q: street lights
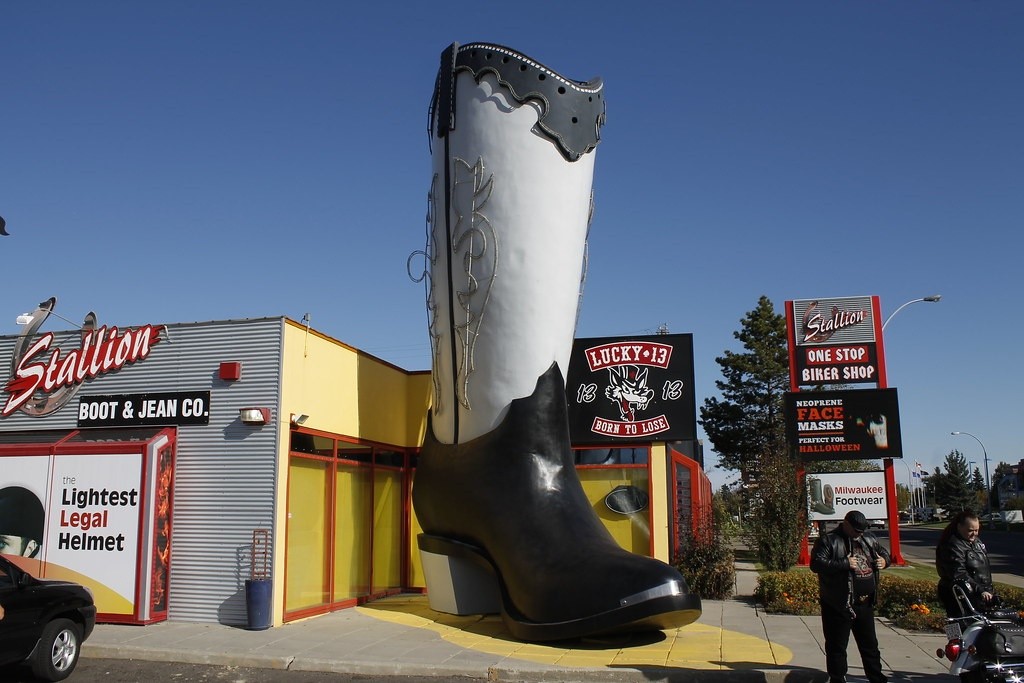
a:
[950,431,993,530]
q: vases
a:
[245,579,272,630]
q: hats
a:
[845,509,869,533]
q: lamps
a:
[15,308,82,330]
[239,407,271,425]
[291,414,310,424]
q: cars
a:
[0,554,97,683]
[896,506,955,524]
[990,512,1002,522]
[867,519,885,530]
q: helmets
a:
[0,485,45,544]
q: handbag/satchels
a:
[843,537,881,608]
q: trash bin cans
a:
[245,579,273,628]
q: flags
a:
[913,462,930,478]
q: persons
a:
[0,487,45,558]
[810,511,891,683]
[936,511,994,633]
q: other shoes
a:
[827,675,848,683]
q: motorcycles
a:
[937,584,1024,683]
